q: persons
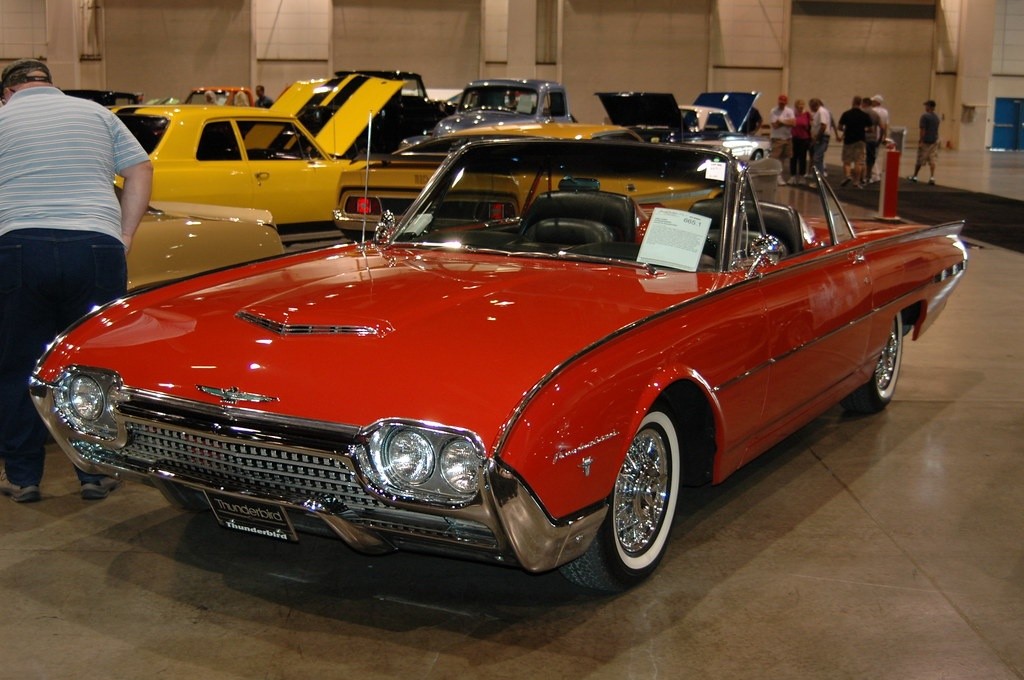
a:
[861,97,887,183]
[0,55,155,505]
[253,84,275,108]
[809,97,842,189]
[767,95,797,186]
[907,100,941,186]
[233,90,251,107]
[837,96,872,192]
[871,94,889,183]
[133,91,146,103]
[204,91,222,105]
[790,98,814,187]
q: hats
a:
[778,95,788,105]
[871,95,884,104]
[923,100,936,107]
[0,58,52,86]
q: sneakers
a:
[0,468,41,502]
[78,475,123,499]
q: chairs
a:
[688,198,804,261]
[516,190,637,244]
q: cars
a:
[63,90,144,106]
[112,180,284,290]
[183,86,254,108]
[102,74,409,234]
[400,77,578,148]
[27,138,967,594]
[334,69,464,155]
[594,90,773,162]
[333,123,721,244]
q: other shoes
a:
[777,175,785,185]
[786,176,796,185]
[839,177,852,187]
[907,175,917,182]
[809,182,818,188]
[928,179,937,185]
[797,177,806,185]
[852,182,863,190]
